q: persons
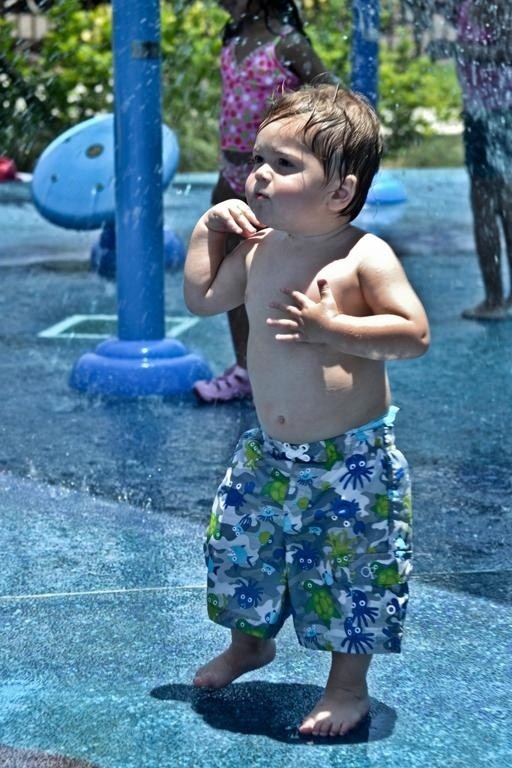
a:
[191,0,330,404]
[402,0,511,320]
[183,86,430,736]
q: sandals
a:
[193,365,250,402]
